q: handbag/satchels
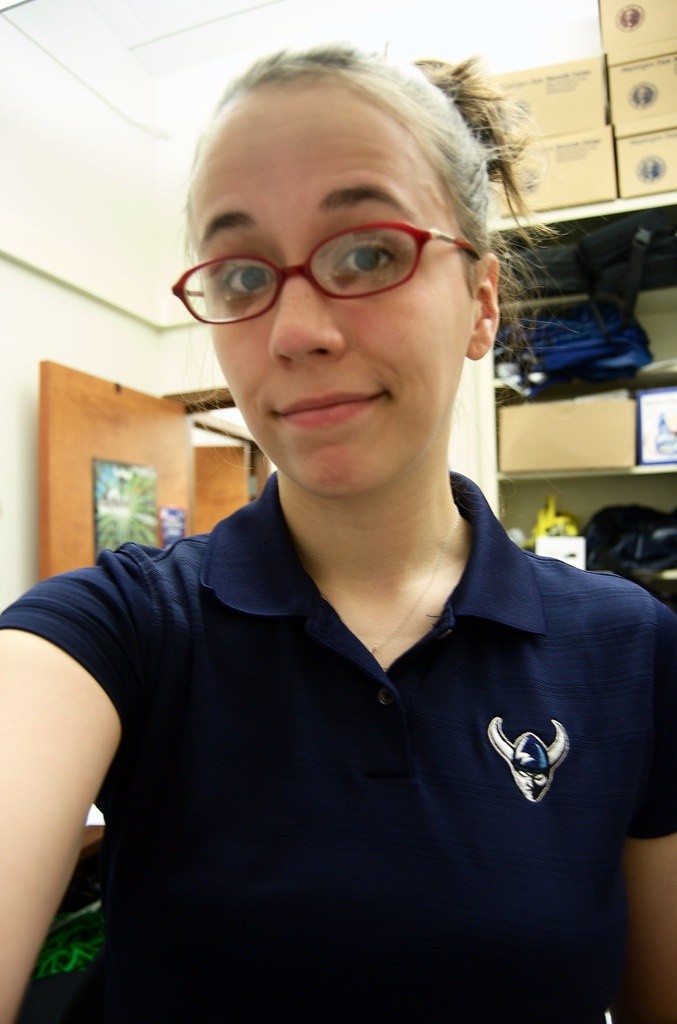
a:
[579,505,677,575]
[499,205,677,333]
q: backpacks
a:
[492,301,652,399]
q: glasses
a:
[172,221,481,325]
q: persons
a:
[0,46,677,1024]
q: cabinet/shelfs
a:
[470,193,677,580]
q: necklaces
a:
[320,505,459,652]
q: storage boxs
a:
[462,0,677,217]
[492,370,677,472]
